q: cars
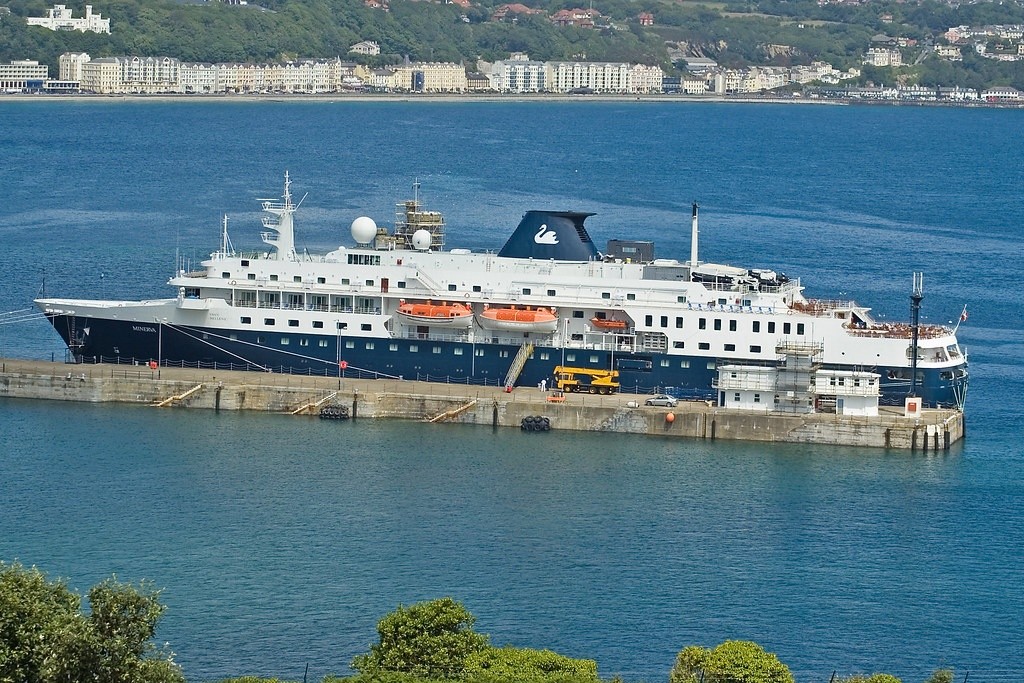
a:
[645,395,679,406]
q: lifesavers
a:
[786,309,794,316]
[566,338,571,345]
[231,280,238,287]
[484,337,491,343]
[463,292,471,298]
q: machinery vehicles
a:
[554,364,620,396]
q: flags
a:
[961,310,968,322]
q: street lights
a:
[469,326,474,385]
[562,318,566,369]
[333,319,339,377]
[153,316,166,369]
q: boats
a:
[739,279,779,290]
[479,309,558,333]
[690,272,736,283]
[32,167,970,412]
[745,269,788,285]
[395,301,474,330]
[590,318,627,329]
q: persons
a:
[541,377,550,391]
